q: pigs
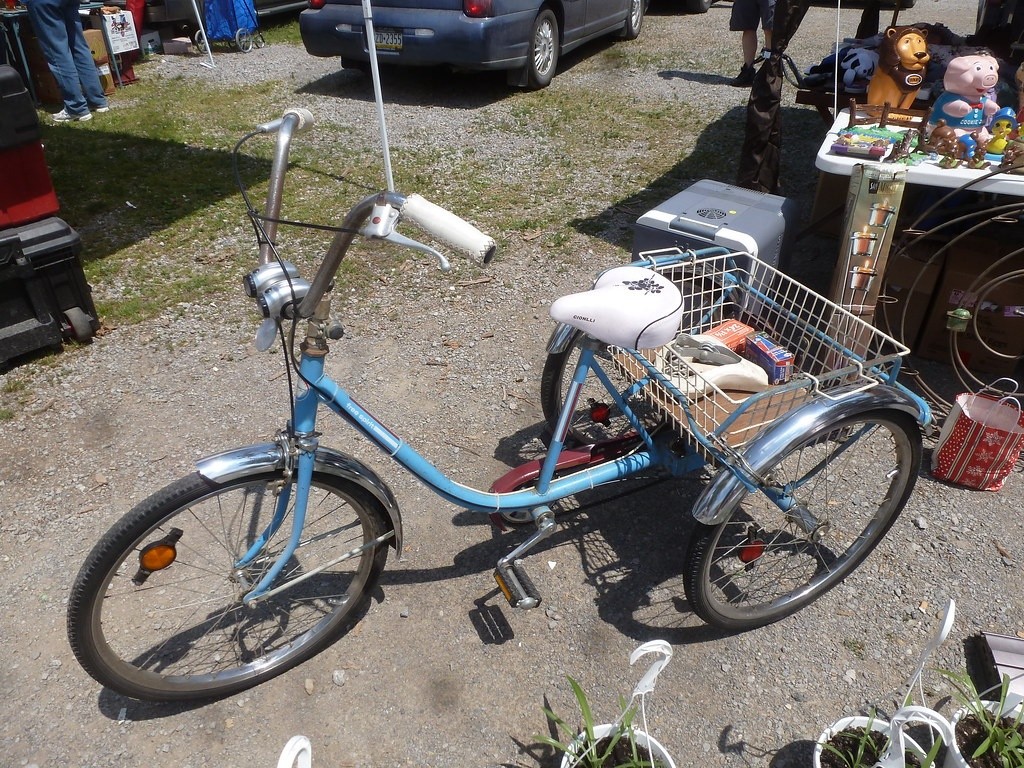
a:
[926,54,999,146]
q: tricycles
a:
[67,107,932,722]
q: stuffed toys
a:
[841,47,880,87]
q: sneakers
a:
[86,103,109,113]
[52,108,92,123]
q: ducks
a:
[986,107,1017,154]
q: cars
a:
[298,0,647,94]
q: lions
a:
[868,27,933,121]
[1000,142,1023,173]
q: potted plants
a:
[932,664,1024,768]
[813,693,936,768]
[531,676,678,768]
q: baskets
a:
[608,252,912,472]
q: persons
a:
[730,0,777,87]
[20,0,109,122]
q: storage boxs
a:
[76,8,139,54]
[916,244,1024,378]
[829,161,909,382]
[162,37,192,55]
[29,28,109,72]
[702,319,754,353]
[139,29,163,57]
[971,630,1024,702]
[870,238,944,353]
[631,180,786,326]
[34,62,116,104]
[744,330,793,386]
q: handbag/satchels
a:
[930,378,1024,492]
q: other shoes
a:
[737,63,755,86]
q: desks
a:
[815,106,1023,195]
[0,2,123,107]
